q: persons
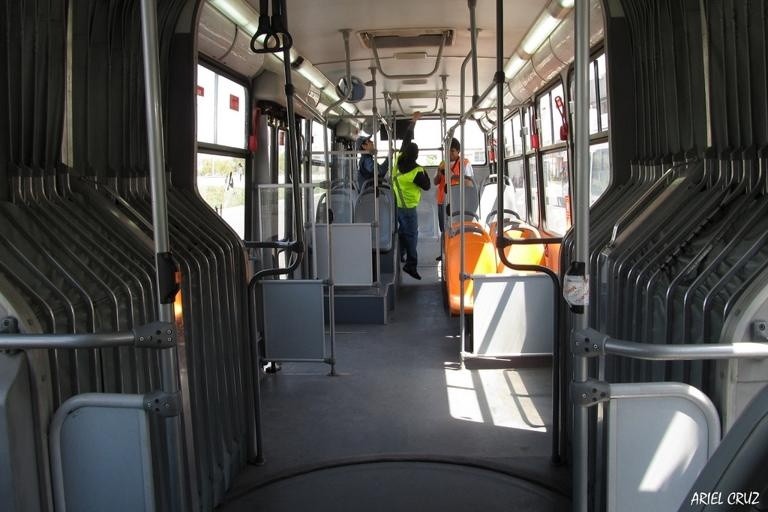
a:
[432,136,474,262]
[354,134,393,193]
[390,110,431,280]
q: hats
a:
[356,135,372,151]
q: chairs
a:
[318,177,394,253]
[444,173,547,315]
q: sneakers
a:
[436,257,442,260]
[403,262,421,280]
[401,255,405,262]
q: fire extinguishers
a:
[489,138,495,160]
